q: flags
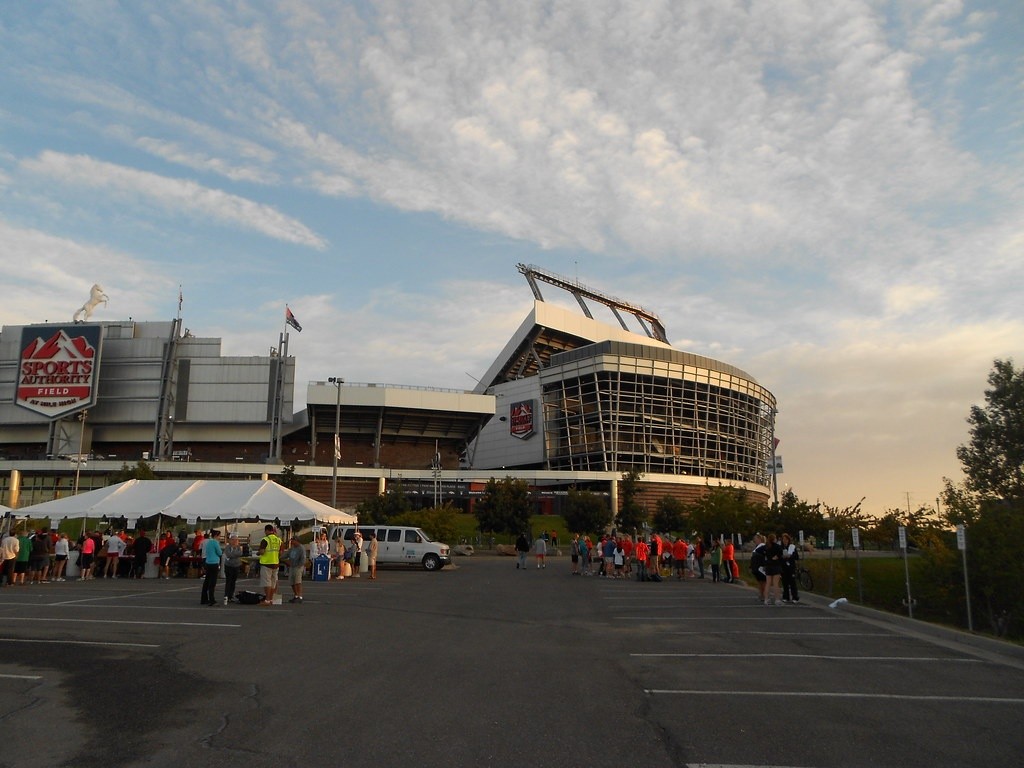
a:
[286,307,302,332]
[178,288,183,311]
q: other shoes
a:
[792,599,799,604]
[762,599,773,605]
[523,567,527,569]
[516,562,520,568]
[5,572,170,586]
[572,569,633,579]
[224,597,235,605]
[352,573,361,578]
[260,600,273,606]
[542,564,545,568]
[336,575,344,580]
[537,565,540,568]
[369,576,376,579]
[698,575,704,579]
[782,599,788,602]
[775,599,785,605]
[288,596,303,603]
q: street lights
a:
[328,377,344,551]
[771,405,778,508]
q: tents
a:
[0,504,27,531]
[0,479,358,581]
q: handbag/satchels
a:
[154,557,160,566]
[343,551,352,560]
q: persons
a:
[514,529,739,584]
[129,530,152,579]
[223,536,243,605]
[334,537,345,579]
[2,527,70,586]
[309,532,328,580]
[76,528,135,580]
[351,532,363,577]
[748,533,800,605]
[157,527,187,580]
[192,528,223,606]
[365,533,378,578]
[256,525,304,607]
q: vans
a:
[327,526,451,571]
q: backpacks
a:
[235,590,265,605]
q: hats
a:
[140,530,145,536]
[289,535,300,542]
[354,532,361,535]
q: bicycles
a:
[793,558,814,592]
